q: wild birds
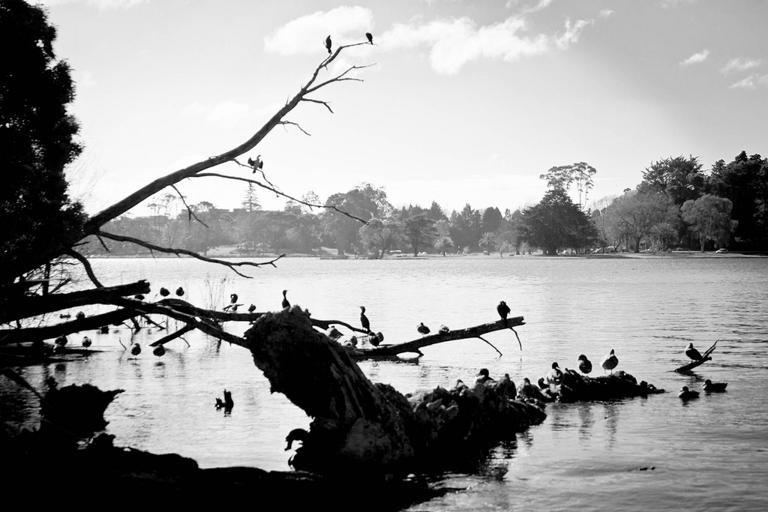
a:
[684,342,702,364]
[79,335,93,350]
[280,289,289,308]
[416,322,448,335]
[228,293,257,312]
[496,301,511,321]
[54,332,69,348]
[160,287,184,298]
[454,349,620,407]
[130,343,164,362]
[327,305,380,349]
[75,310,84,321]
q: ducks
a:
[678,379,729,400]
